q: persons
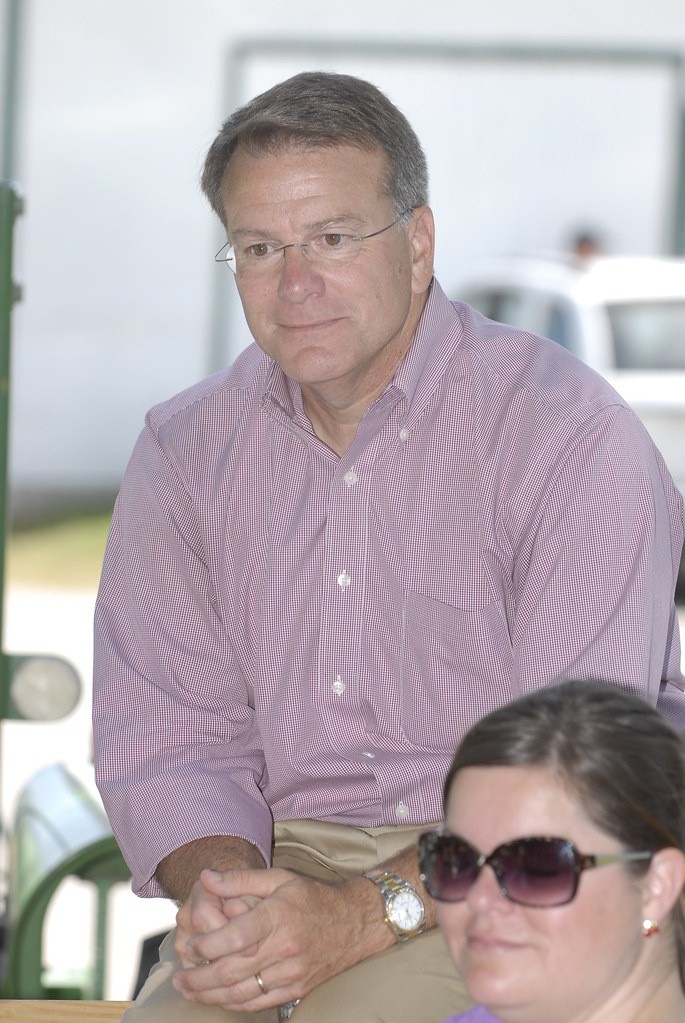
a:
[434,682,685,1023]
[92,75,685,1023]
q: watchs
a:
[364,868,425,943]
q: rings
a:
[255,973,267,994]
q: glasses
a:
[216,211,404,275]
[419,828,652,906]
